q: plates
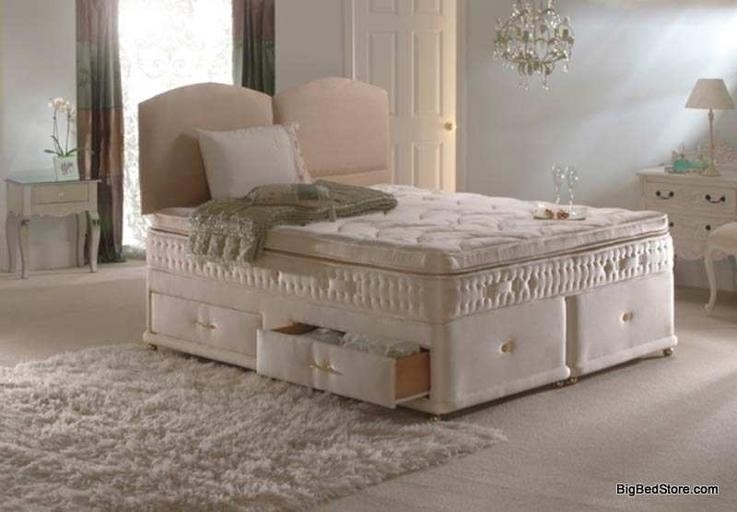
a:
[535,207,588,220]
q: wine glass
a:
[551,165,581,215]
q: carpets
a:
[0,332,511,511]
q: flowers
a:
[44,93,81,160]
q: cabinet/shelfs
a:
[4,173,102,277]
[634,165,737,263]
[144,285,431,412]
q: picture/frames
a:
[56,153,81,181]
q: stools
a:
[702,221,736,314]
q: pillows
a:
[192,119,309,204]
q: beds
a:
[138,70,678,420]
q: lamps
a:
[488,0,576,91]
[685,78,736,178]
